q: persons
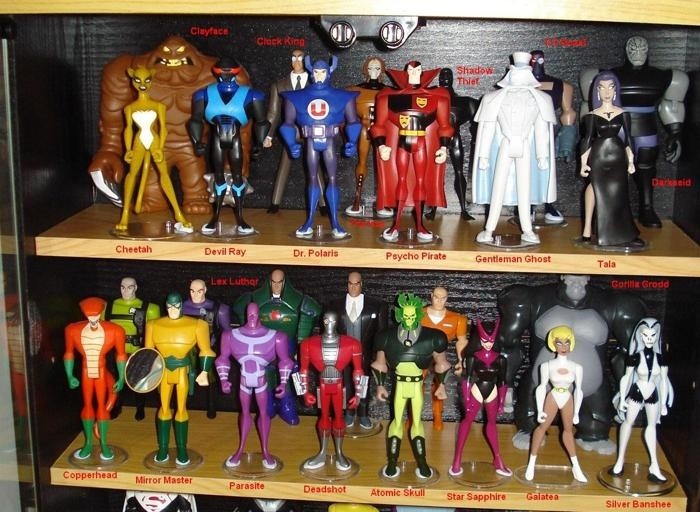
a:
[62,274,678,512]
[187,36,690,250]
[63,274,673,482]
[86,35,691,255]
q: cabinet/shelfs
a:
[1,3,700,511]
